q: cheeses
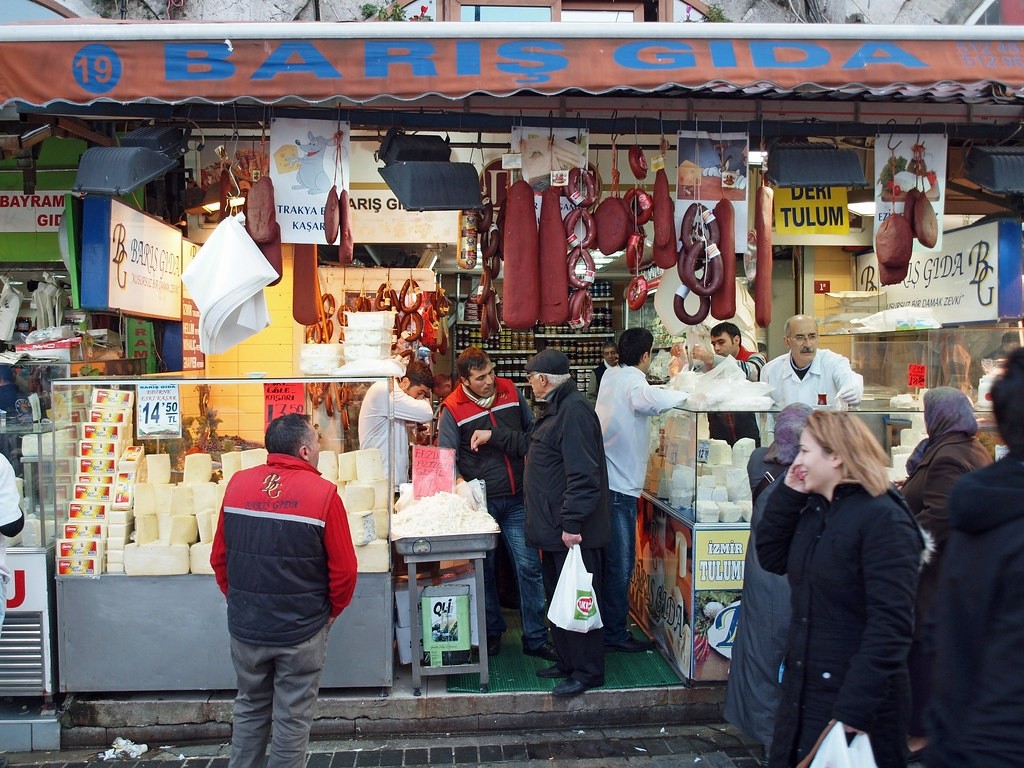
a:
[886,388,931,482]
[122,448,268,574]
[316,449,390,573]
[648,412,756,524]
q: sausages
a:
[306,279,450,429]
[564,167,600,328]
[673,203,725,326]
[476,194,504,339]
[625,147,654,312]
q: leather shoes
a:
[537,662,571,678]
[603,632,657,653]
[552,676,606,697]
[472,635,499,656]
[522,639,560,662]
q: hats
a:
[524,349,570,375]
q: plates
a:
[708,601,740,658]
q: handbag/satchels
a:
[547,542,607,631]
[797,721,878,768]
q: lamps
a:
[764,137,870,189]
[963,143,1024,193]
[71,119,192,199]
[376,126,486,211]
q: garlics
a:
[703,601,724,617]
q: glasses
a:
[788,334,818,342]
[525,371,539,383]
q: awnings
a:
[0,14,1024,117]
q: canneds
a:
[456,279,613,391]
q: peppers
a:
[694,629,709,662]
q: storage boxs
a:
[15,342,79,363]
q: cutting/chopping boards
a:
[880,171,939,202]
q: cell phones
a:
[796,467,804,481]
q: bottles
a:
[977,375,995,407]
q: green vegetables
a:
[695,591,743,634]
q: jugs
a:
[907,145,933,176]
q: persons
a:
[469,347,611,697]
[761,314,866,410]
[721,349,1024,768]
[0,365,29,476]
[436,345,558,662]
[355,358,434,494]
[596,326,688,653]
[0,450,24,768]
[210,413,359,768]
[434,373,462,397]
[670,322,766,448]
[588,341,619,410]
